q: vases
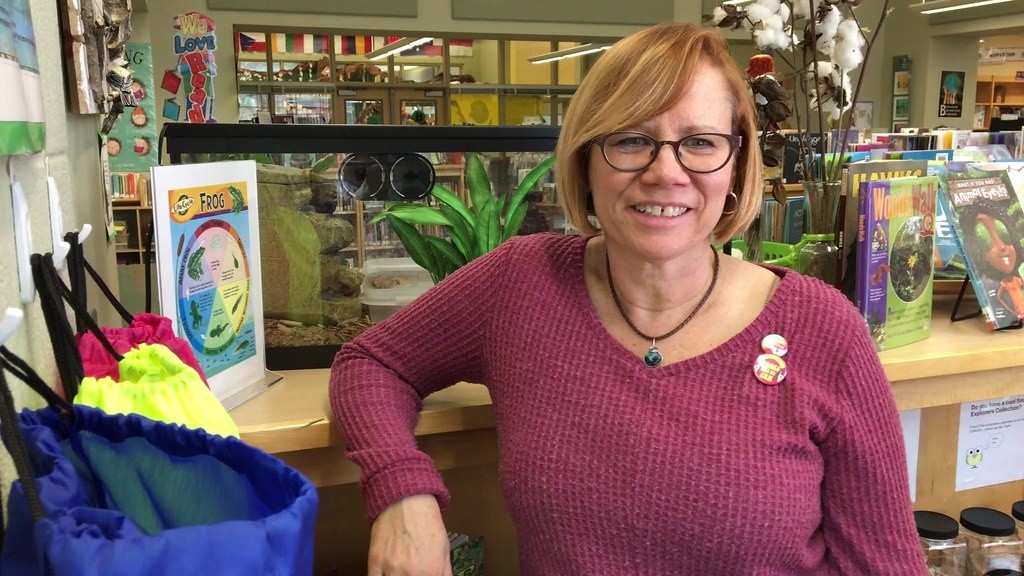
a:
[800,179,842,291]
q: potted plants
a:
[364,156,559,287]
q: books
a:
[755,128,1024,354]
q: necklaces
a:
[606,243,719,367]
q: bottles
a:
[913,500,1024,576]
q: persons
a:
[330,22,931,576]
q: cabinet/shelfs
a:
[104,175,157,264]
[972,75,1023,134]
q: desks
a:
[220,279,1022,576]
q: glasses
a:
[592,130,742,174]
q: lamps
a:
[527,43,614,65]
[364,37,433,61]
[909,1,1012,16]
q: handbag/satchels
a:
[1,344,318,576]
[63,231,212,393]
[28,252,242,440]
